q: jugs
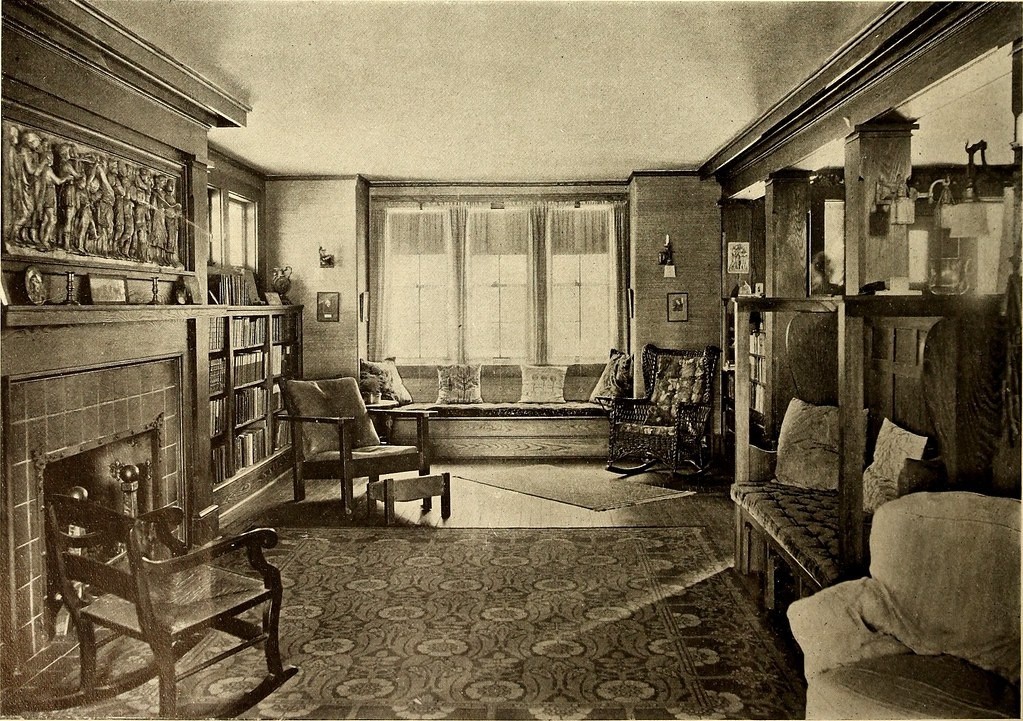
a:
[924,256,972,296]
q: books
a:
[206,272,251,307]
[232,316,269,348]
[207,315,227,350]
[272,344,296,378]
[208,356,227,394]
[272,382,286,412]
[274,416,294,452]
[235,387,267,428]
[271,315,299,342]
[210,397,226,436]
[211,440,230,484]
[232,420,268,476]
[233,349,268,387]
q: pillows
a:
[519,363,566,404]
[862,417,929,512]
[361,358,414,404]
[435,362,483,405]
[776,398,868,489]
[588,347,630,407]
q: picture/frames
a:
[667,292,689,322]
[318,292,339,322]
[86,272,128,306]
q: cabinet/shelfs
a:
[204,305,300,526]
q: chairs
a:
[277,376,435,515]
[46,465,299,719]
[785,491,1021,720]
[595,341,722,476]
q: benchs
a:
[732,481,872,612]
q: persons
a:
[10,130,182,266]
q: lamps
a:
[319,245,334,268]
[875,137,1022,237]
[659,236,677,280]
